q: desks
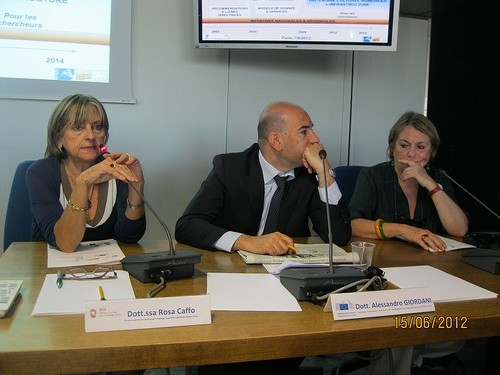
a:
[0,236,500,375]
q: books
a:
[237,243,366,264]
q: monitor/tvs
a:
[193,0,401,53]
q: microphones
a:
[279,149,378,303]
[430,164,500,274]
[99,144,202,284]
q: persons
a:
[175,99,353,375]
[26,93,147,253]
[347,110,469,375]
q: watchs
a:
[430,183,444,195]
[316,167,336,181]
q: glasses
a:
[62,264,118,280]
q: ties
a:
[263,175,291,234]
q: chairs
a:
[4,160,38,251]
[330,166,371,202]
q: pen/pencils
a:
[286,244,300,252]
[98,285,106,300]
[57,271,63,288]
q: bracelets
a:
[375,219,387,240]
[127,198,145,209]
[68,199,92,210]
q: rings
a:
[422,234,428,239]
[109,164,114,168]
